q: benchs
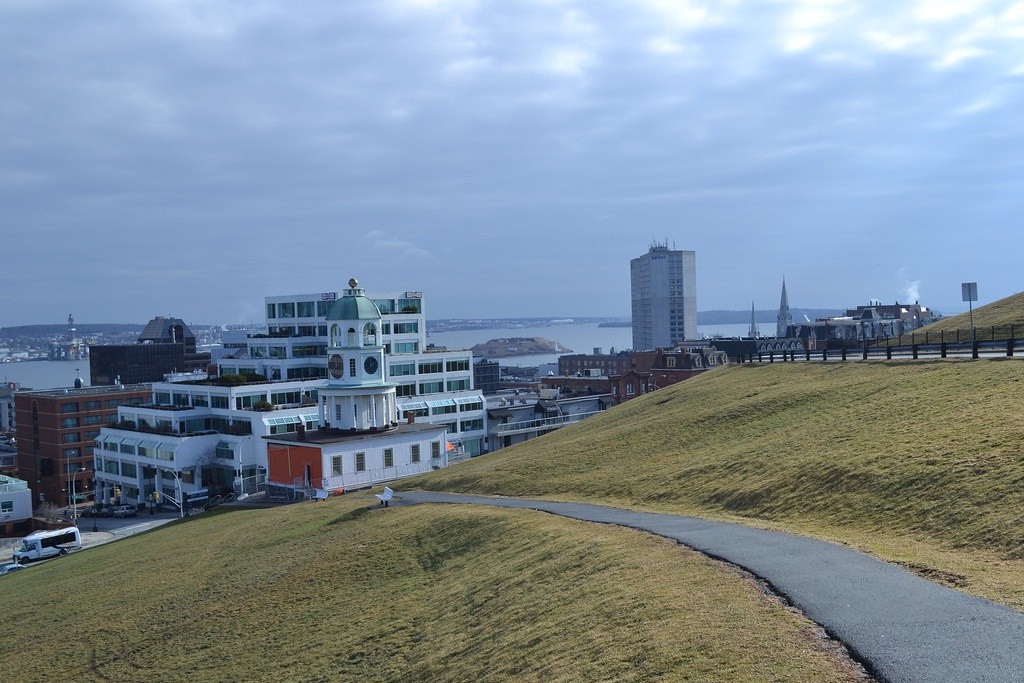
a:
[312,490,329,502]
[375,486,394,508]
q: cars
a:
[0,563,27,576]
[113,505,137,519]
[96,508,113,517]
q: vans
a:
[12,527,82,561]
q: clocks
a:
[363,356,379,375]
[328,354,343,380]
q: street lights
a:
[73,467,86,527]
[239,436,253,496]
[164,468,184,518]
